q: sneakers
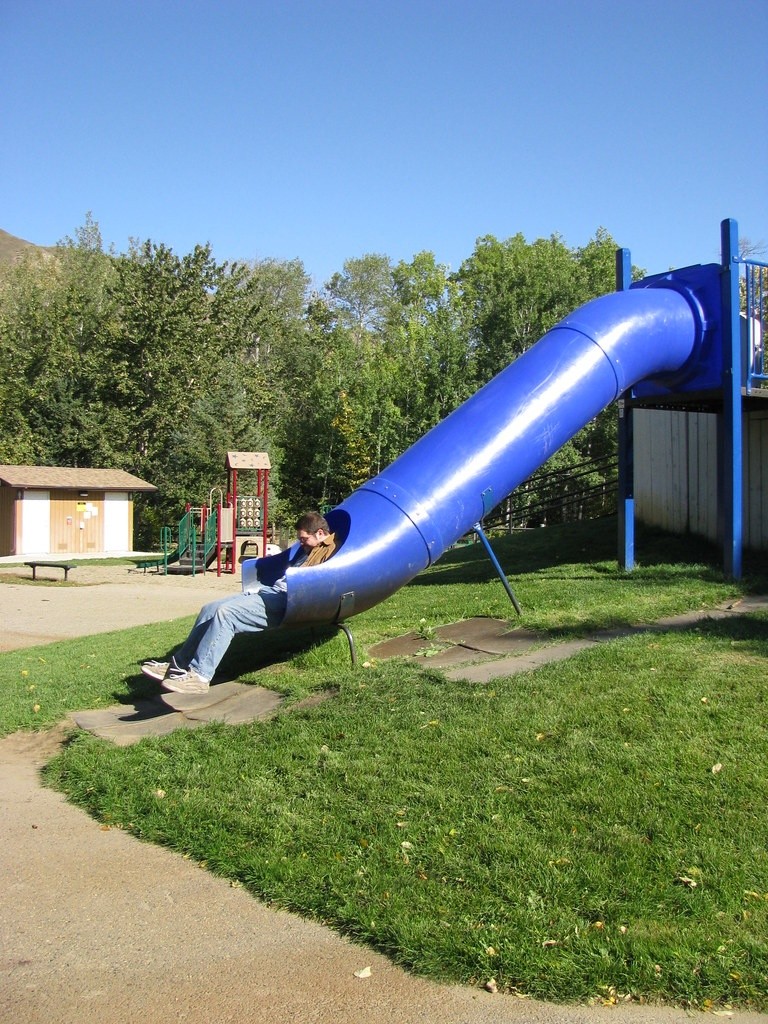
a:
[141,656,210,694]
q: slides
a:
[238,263,734,639]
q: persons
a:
[140,512,344,695]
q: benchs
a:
[24,561,77,581]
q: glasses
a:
[300,528,323,543]
